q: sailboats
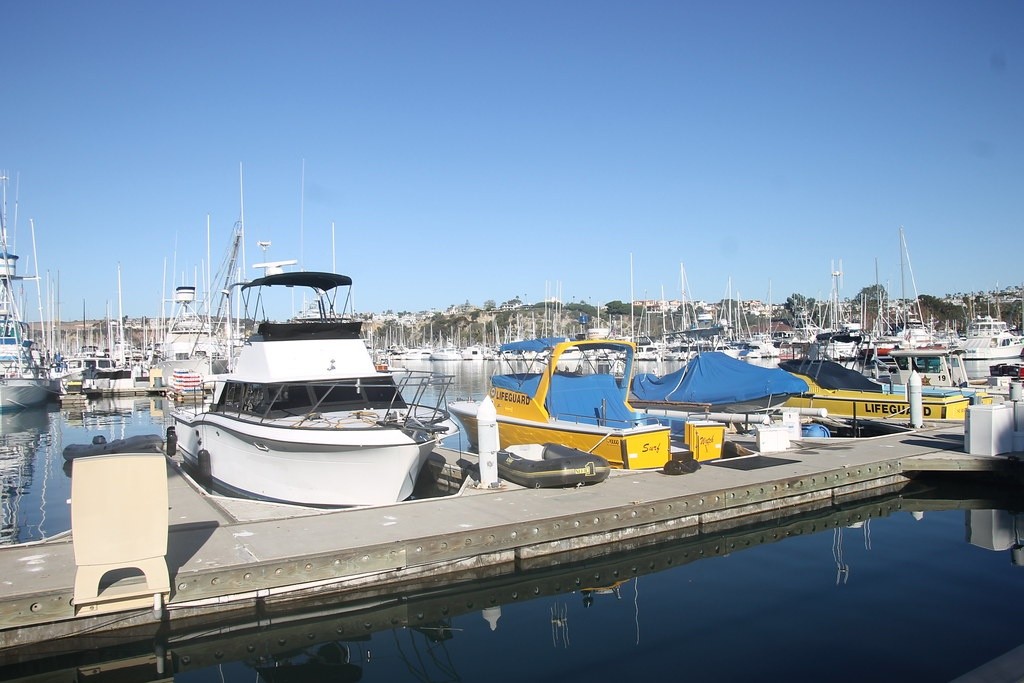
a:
[0,160,969,414]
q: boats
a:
[627,351,810,416]
[446,333,726,476]
[777,357,994,420]
[500,442,611,489]
[167,161,459,510]
[953,297,1024,360]
